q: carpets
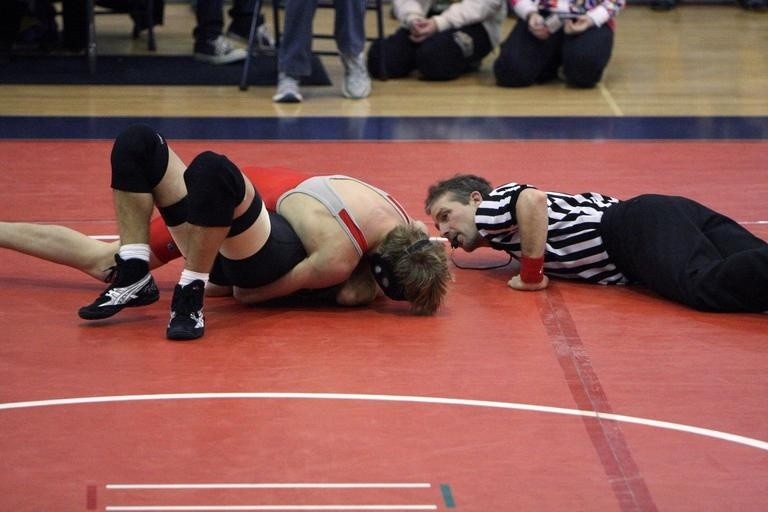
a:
[3,48,333,86]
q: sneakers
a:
[274,72,302,102]
[338,51,372,99]
[194,35,247,65]
[79,254,160,320]
[167,279,205,339]
[227,20,274,47]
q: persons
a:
[366,0,508,80]
[271,0,373,102]
[0,166,448,307]
[192,0,276,65]
[79,124,384,340]
[1,1,96,64]
[492,1,627,87]
[424,175,767,310]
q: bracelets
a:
[519,255,544,284]
